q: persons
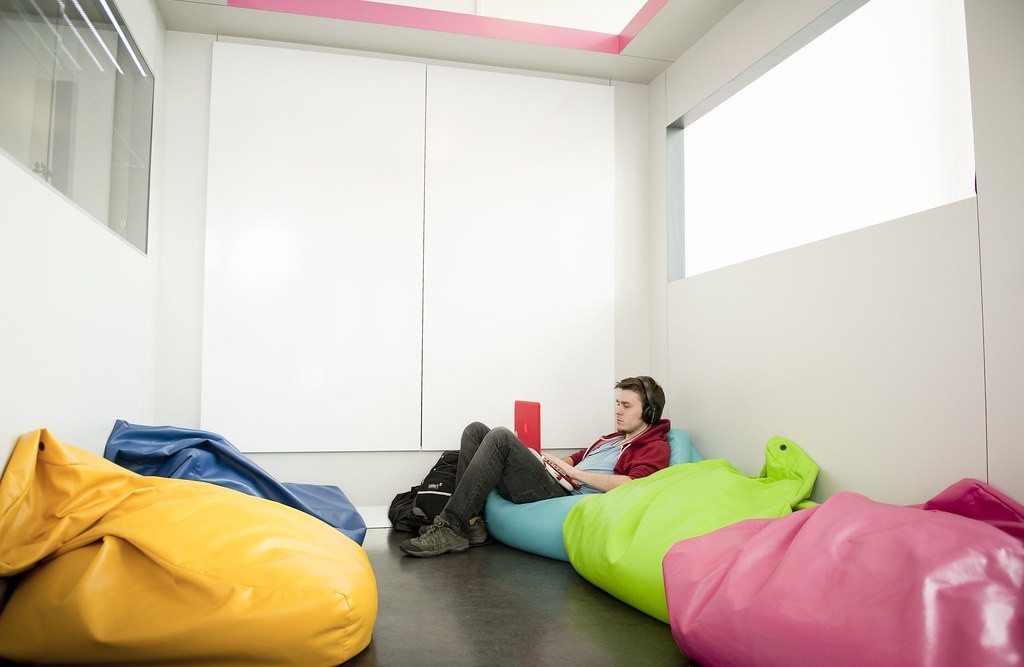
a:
[399,375,670,556]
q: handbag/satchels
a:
[388,485,421,532]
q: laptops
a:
[513,400,581,489]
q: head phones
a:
[637,375,663,425]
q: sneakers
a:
[399,515,470,557]
[467,519,487,544]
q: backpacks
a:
[412,449,459,525]
